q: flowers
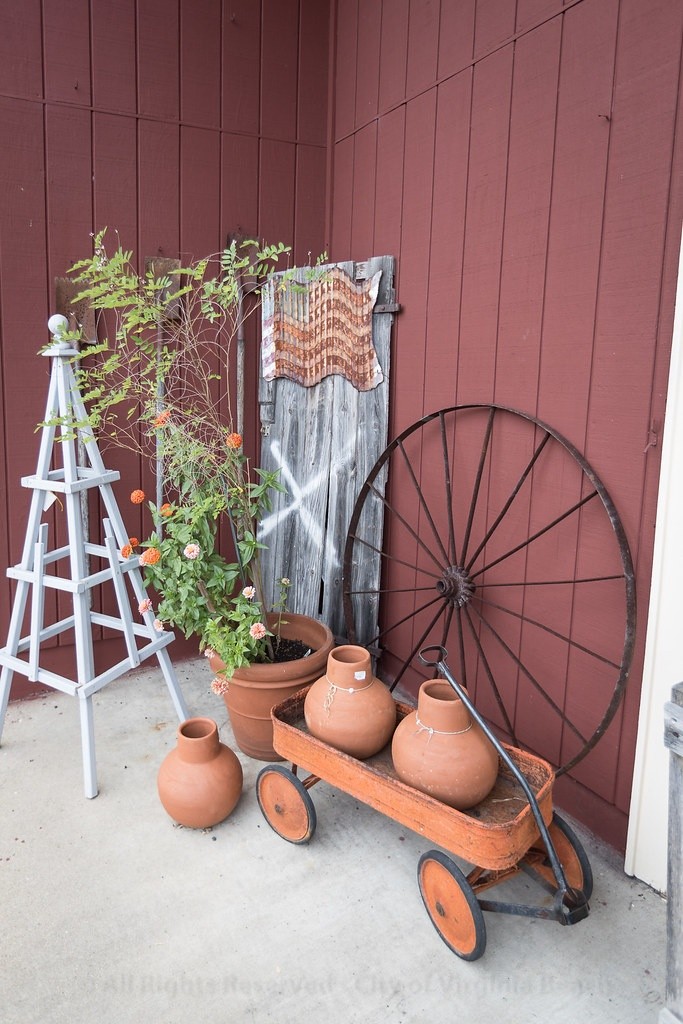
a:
[113,397,269,696]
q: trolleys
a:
[255,683,593,964]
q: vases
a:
[155,719,245,831]
[392,680,498,811]
[305,642,398,759]
[208,610,336,766]
